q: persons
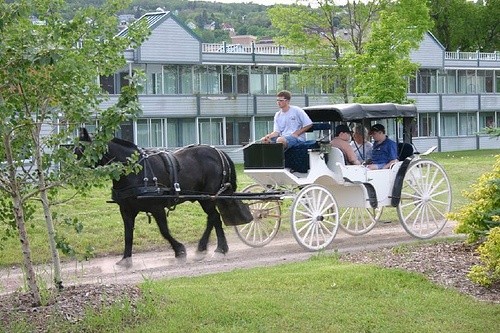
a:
[330,124,362,164]
[260,90,314,154]
[366,123,398,170]
[350,125,373,163]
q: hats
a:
[368,124,384,134]
[336,125,353,133]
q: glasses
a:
[277,98,287,102]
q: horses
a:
[74,127,237,266]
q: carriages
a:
[63,101,452,269]
